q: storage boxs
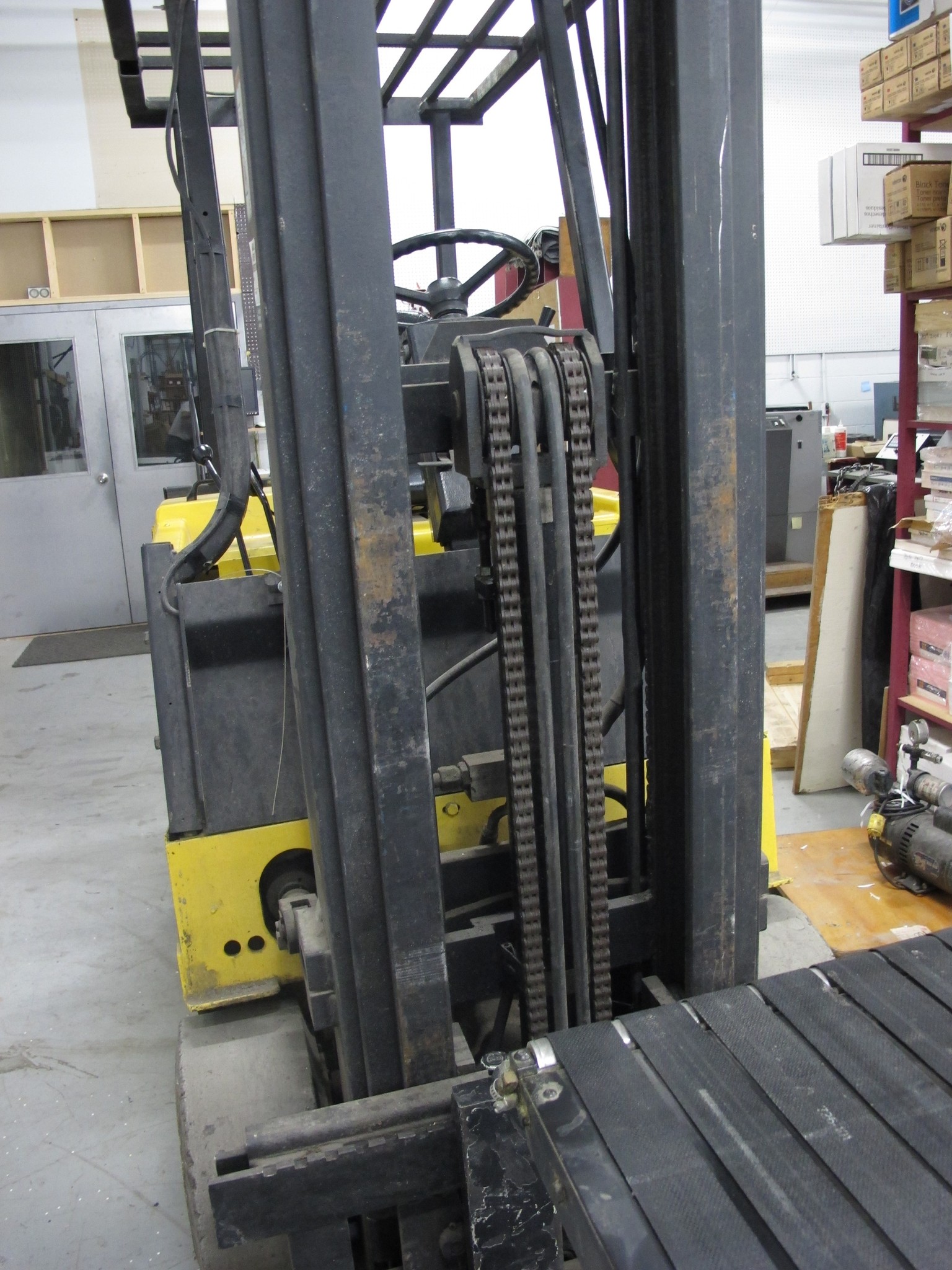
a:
[859,0,952,121]
[819,143,952,293]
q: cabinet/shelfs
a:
[887,113,952,807]
